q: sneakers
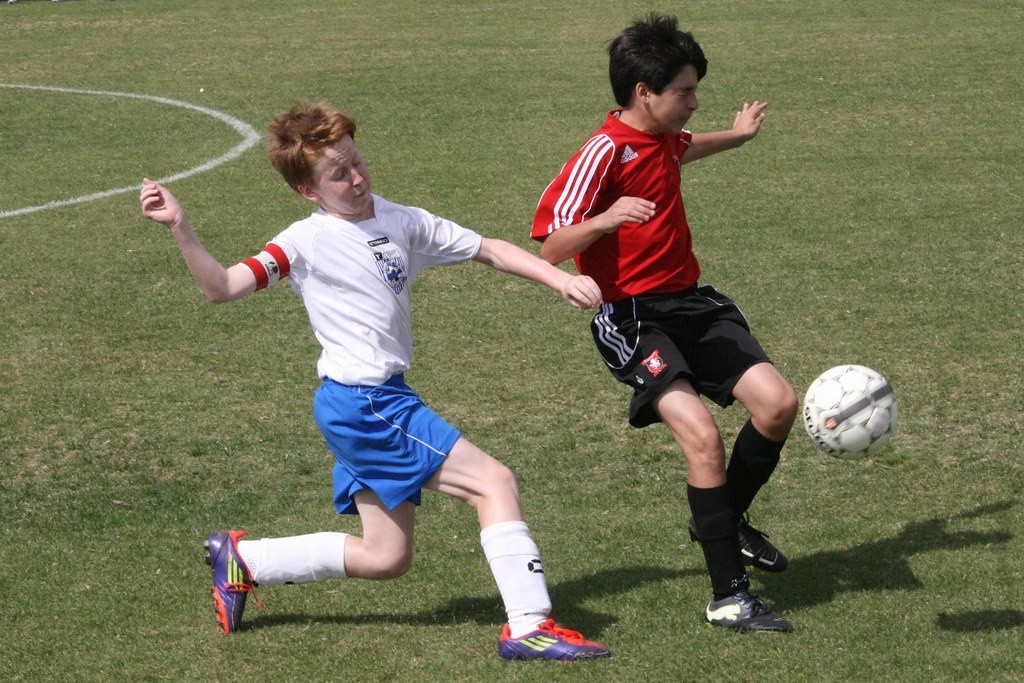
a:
[203,531,262,635]
[496,619,611,664]
[688,511,789,572]
[704,591,794,633]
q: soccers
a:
[803,361,897,461]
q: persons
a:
[138,102,612,661]
[529,13,797,637]
